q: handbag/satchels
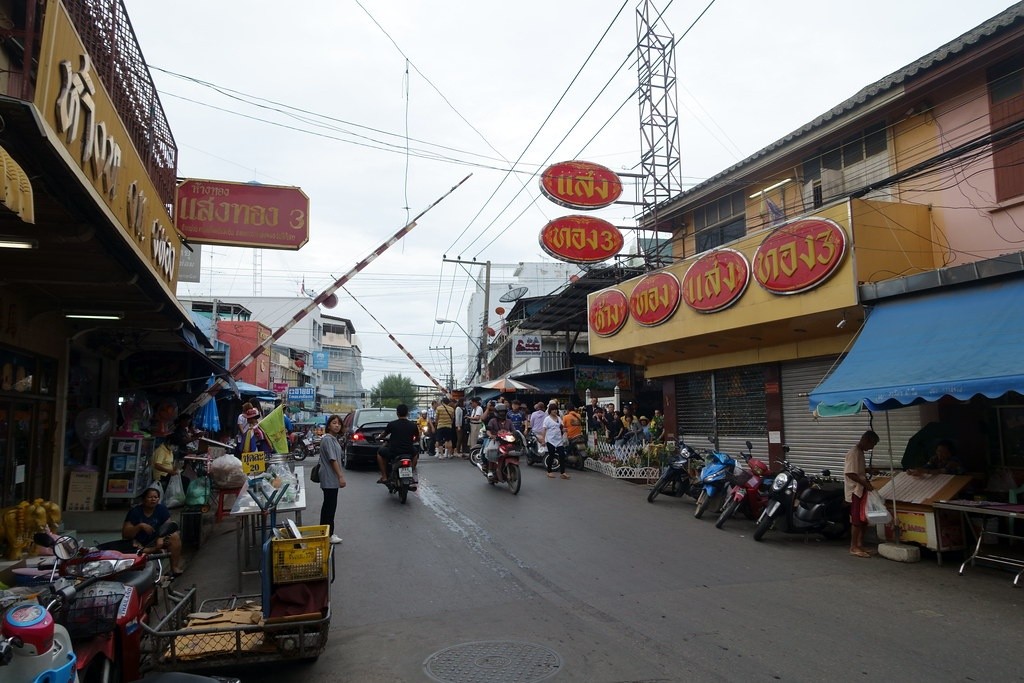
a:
[209,454,247,488]
[310,463,321,484]
[484,437,498,462]
[185,476,210,505]
[149,481,164,504]
[164,473,186,508]
[860,488,893,525]
[562,432,570,447]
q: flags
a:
[259,405,288,453]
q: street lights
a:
[435,317,487,384]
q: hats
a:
[638,416,649,422]
[242,409,260,419]
[534,402,546,412]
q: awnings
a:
[234,380,276,396]
[799,275,1024,545]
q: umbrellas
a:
[474,377,540,399]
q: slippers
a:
[377,478,387,483]
[409,477,415,484]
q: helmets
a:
[493,403,508,419]
[421,410,428,419]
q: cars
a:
[337,408,399,471]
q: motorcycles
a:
[375,436,419,505]
[476,428,525,495]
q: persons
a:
[584,397,665,445]
[121,488,183,575]
[173,413,208,473]
[542,404,570,478]
[375,404,420,483]
[417,410,427,431]
[317,415,346,544]
[455,398,583,472]
[275,399,293,431]
[906,440,981,499]
[241,409,272,454]
[150,433,189,490]
[843,431,879,558]
[237,402,252,441]
[435,398,457,459]
[427,401,438,456]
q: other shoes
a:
[488,472,494,479]
[849,549,870,558]
[419,444,471,460]
[858,547,872,553]
[329,534,343,543]
[547,472,556,478]
[560,473,570,479]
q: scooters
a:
[647,428,777,529]
[752,444,851,544]
[0,519,244,683]
[469,422,589,474]
[281,425,324,462]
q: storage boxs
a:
[271,524,330,582]
[65,470,101,513]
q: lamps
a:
[64,309,128,320]
[0,234,39,249]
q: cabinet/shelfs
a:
[101,435,156,511]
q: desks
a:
[229,462,307,575]
[936,499,1024,588]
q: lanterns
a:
[495,307,504,315]
[489,328,494,336]
[296,360,304,368]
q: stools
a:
[216,486,242,524]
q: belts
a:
[470,422,483,424]
